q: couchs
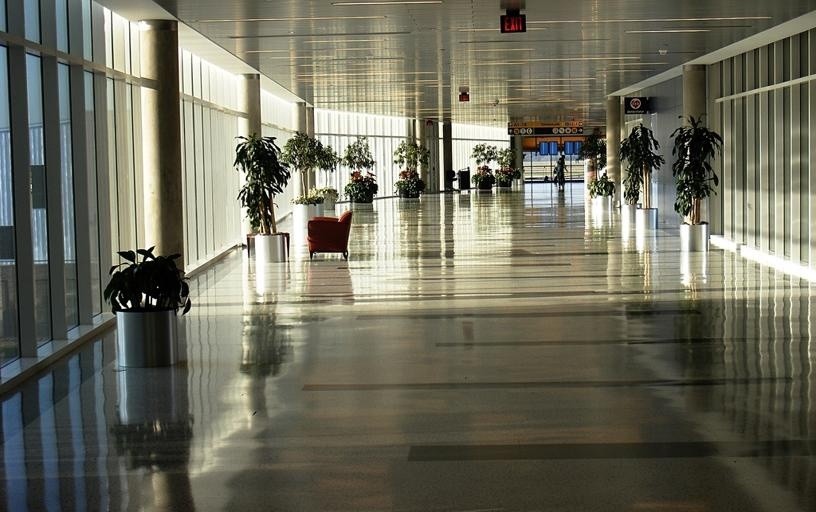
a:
[307,208,353,261]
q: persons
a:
[555,155,569,192]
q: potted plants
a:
[233,132,290,261]
[281,129,520,220]
[586,170,618,211]
[616,122,661,230]
[666,112,726,253]
[102,246,193,369]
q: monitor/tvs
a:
[564,141,573,155]
[549,141,558,155]
[540,142,548,155]
[574,141,583,155]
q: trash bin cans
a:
[458,167,470,190]
